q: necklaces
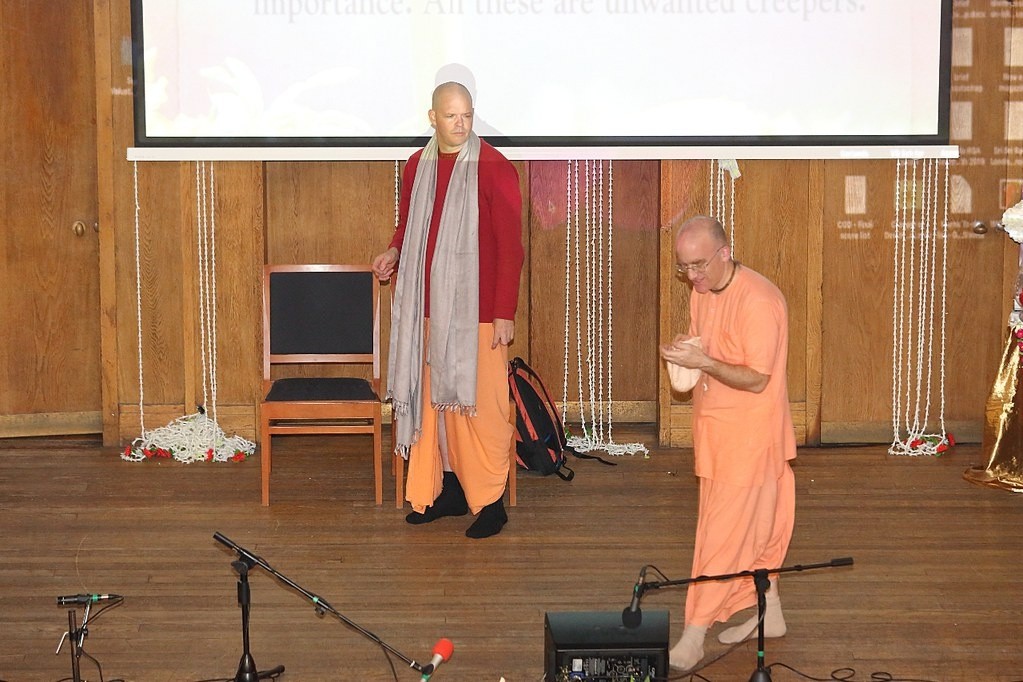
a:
[709,259,738,292]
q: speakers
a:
[543,611,671,682]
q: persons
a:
[660,214,797,669]
[370,82,525,539]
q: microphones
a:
[420,638,454,682]
[58,593,119,605]
[621,566,647,628]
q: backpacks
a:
[507,356,568,475]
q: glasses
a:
[674,243,726,273]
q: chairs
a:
[391,402,519,507]
[259,262,384,506]
